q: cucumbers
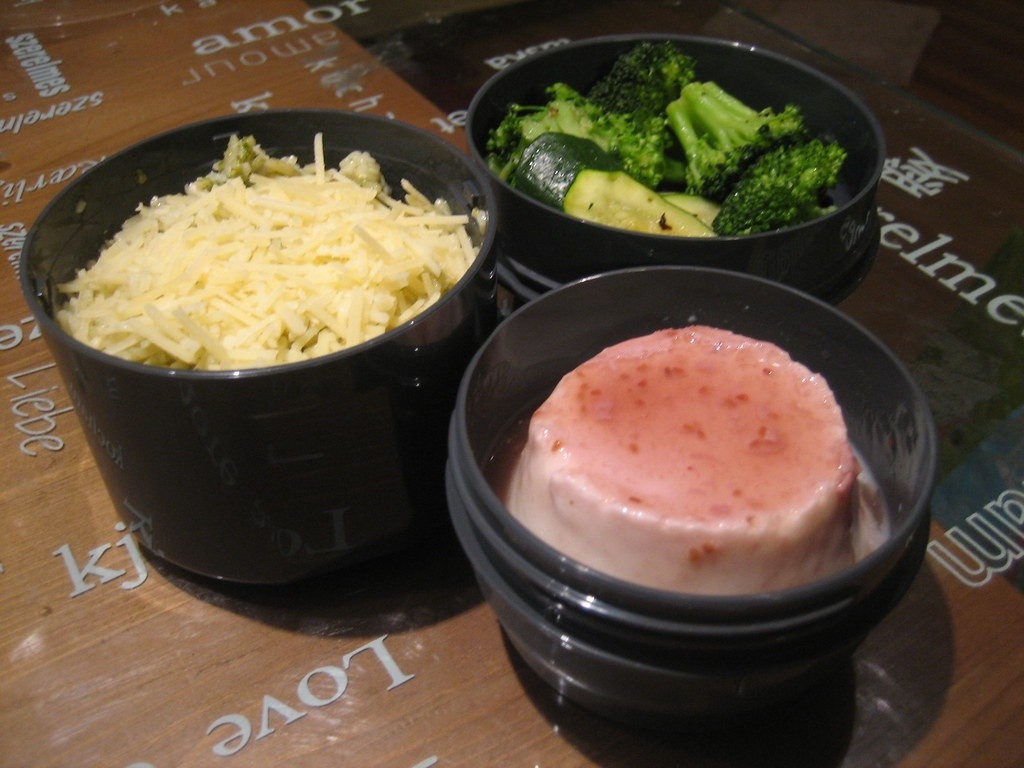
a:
[514,133,718,237]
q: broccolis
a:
[486,39,848,238]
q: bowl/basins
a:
[20,107,490,586]
[464,37,881,300]
[445,267,938,721]
